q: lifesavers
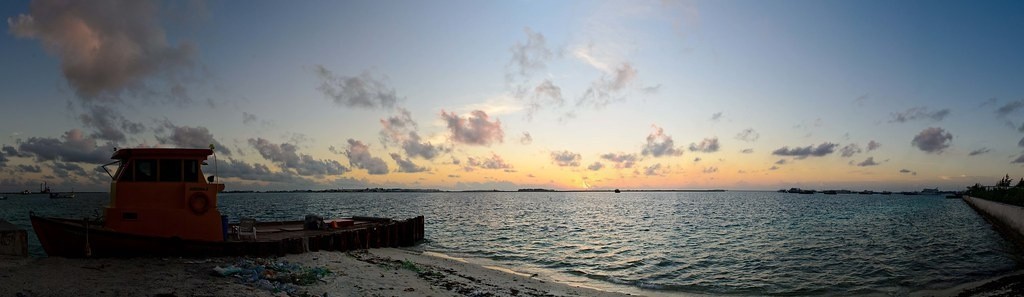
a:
[189,192,210,213]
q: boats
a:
[0,197,8,200]
[27,147,426,258]
[49,191,75,198]
[24,190,31,195]
